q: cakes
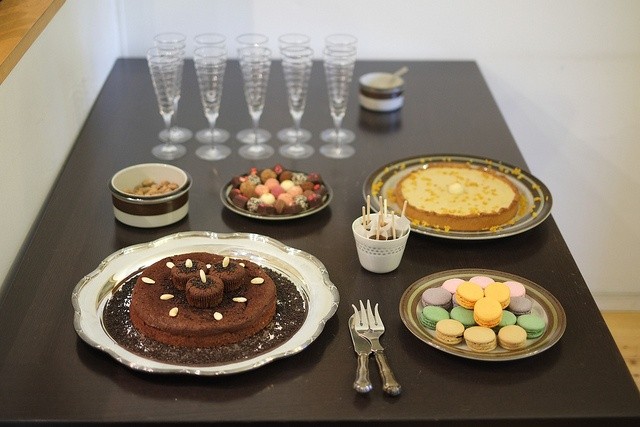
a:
[396,165,520,231]
[129,252,278,348]
[229,163,327,215]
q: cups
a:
[352,214,412,272]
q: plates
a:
[363,154,553,241]
[71,230,341,383]
[398,266,569,361]
[220,167,333,220]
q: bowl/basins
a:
[358,71,406,113]
[110,163,192,231]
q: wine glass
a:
[236,31,272,145]
[278,34,311,142]
[321,45,356,159]
[149,31,191,144]
[237,47,274,161]
[147,48,185,160]
[321,34,356,143]
[195,47,231,161]
[193,28,228,143]
[281,46,316,160]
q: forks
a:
[352,298,403,396]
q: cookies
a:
[420,276,545,353]
